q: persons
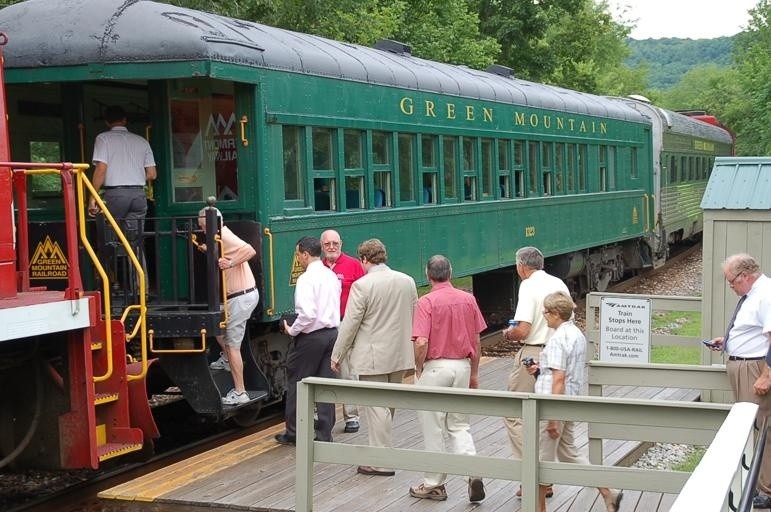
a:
[314,229,361,432]
[525,292,624,512]
[330,237,418,477]
[707,254,771,508]
[502,246,573,498]
[196,206,259,406]
[408,255,488,502]
[285,142,329,194]
[87,105,158,315]
[275,235,343,447]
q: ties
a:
[720,295,747,356]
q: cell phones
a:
[703,340,719,348]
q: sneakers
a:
[468,476,485,501]
[410,483,447,501]
[221,388,250,405]
[275,434,296,444]
[517,484,553,498]
[210,351,231,372]
[358,465,395,476]
[604,489,623,512]
[345,421,359,432]
[753,489,771,509]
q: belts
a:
[519,343,545,348]
[227,285,257,299]
[103,185,144,190]
[729,355,765,361]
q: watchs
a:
[228,259,234,269]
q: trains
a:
[0,1,737,473]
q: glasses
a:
[728,271,744,285]
[325,242,340,246]
